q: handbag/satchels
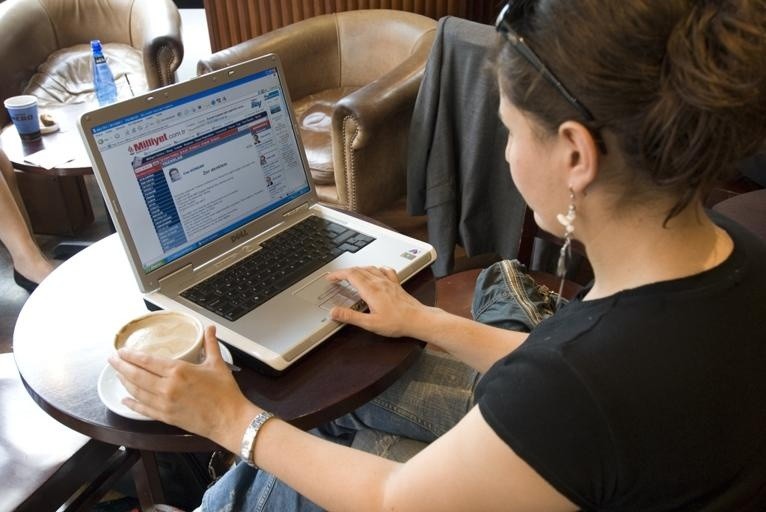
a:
[471,259,570,333]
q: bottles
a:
[89,40,118,109]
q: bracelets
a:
[239,410,278,469]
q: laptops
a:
[76,51,437,371]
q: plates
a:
[97,341,234,421]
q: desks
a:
[13,208,436,451]
[0,106,116,257]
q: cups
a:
[4,95,42,144]
[114,311,205,361]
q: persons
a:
[259,155,267,167]
[106,0,766,512]
[168,167,182,182]
[252,132,262,146]
[264,176,274,187]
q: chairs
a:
[406,17,594,269]
[0,0,183,128]
[197,9,438,216]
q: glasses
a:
[494,0,609,156]
